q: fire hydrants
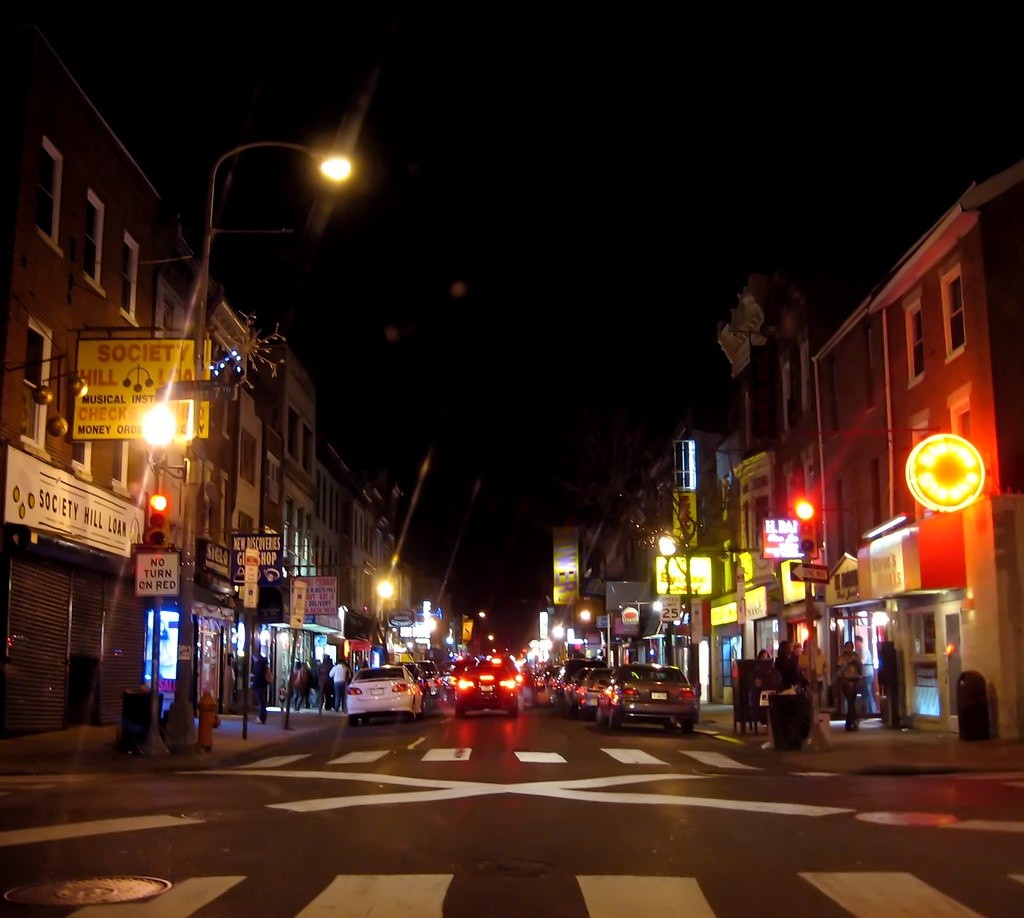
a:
[194,690,221,754]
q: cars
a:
[396,651,614,715]
[349,664,424,727]
[454,664,522,716]
[596,663,701,734]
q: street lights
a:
[168,142,352,745]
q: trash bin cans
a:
[116,685,165,754]
[957,669,991,741]
[766,693,812,753]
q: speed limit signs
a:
[660,595,681,621]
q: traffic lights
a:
[145,492,172,549]
[794,496,817,561]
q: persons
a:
[775,640,827,707]
[252,653,267,724]
[287,658,353,711]
[757,649,770,659]
[836,641,862,729]
[855,636,877,712]
[356,655,369,669]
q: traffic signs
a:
[790,562,831,585]
